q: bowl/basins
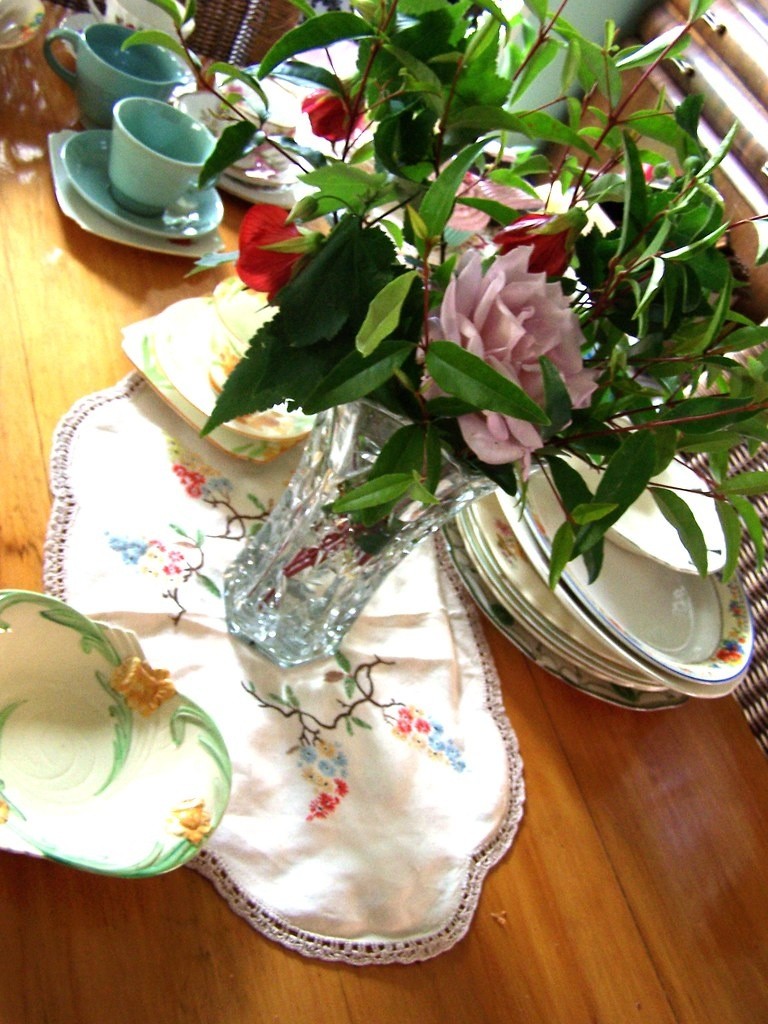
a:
[0,0,45,49]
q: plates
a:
[57,12,196,103]
[174,91,323,211]
[119,318,313,465]
[440,414,755,713]
[148,296,316,443]
[0,587,232,879]
[48,129,223,258]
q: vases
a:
[227,395,500,667]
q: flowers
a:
[120,1,768,608]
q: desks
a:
[0,1,768,1024]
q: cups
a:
[204,280,279,414]
[88,0,194,45]
[219,81,297,171]
[42,23,189,129]
[108,96,221,219]
